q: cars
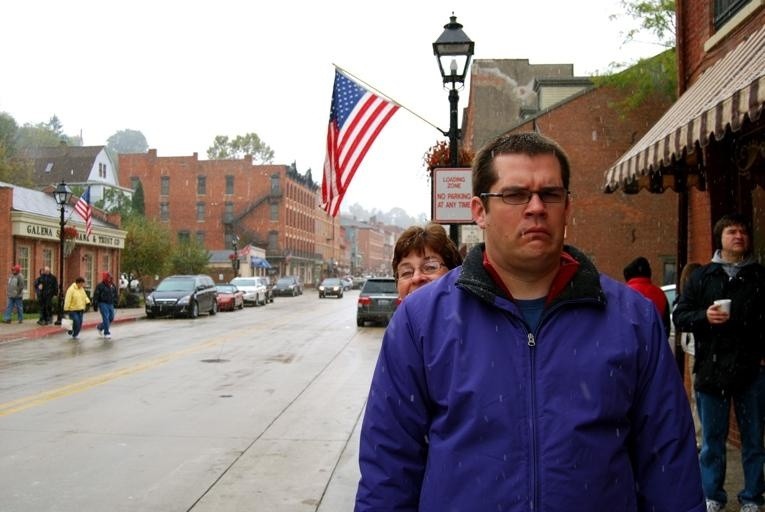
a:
[318,278,343,298]
[119,272,139,290]
[341,274,376,291]
[215,283,244,311]
[658,283,677,355]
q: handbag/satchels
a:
[61,311,74,331]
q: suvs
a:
[253,276,275,303]
[229,277,266,306]
[357,277,402,326]
[271,276,303,297]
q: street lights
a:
[53,179,72,327]
[430,11,476,257]
[319,259,323,281]
[231,232,240,278]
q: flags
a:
[320,71,400,220]
[76,187,93,240]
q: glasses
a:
[480,187,570,205]
[393,261,449,281]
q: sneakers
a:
[96,325,102,336]
[37,319,61,325]
[740,503,760,512]
[705,497,725,512]
[104,334,112,339]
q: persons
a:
[392,225,461,303]
[0,262,117,339]
[672,214,764,511]
[624,256,670,340]
[353,133,703,511]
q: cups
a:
[714,297,732,320]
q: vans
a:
[143,274,219,318]
